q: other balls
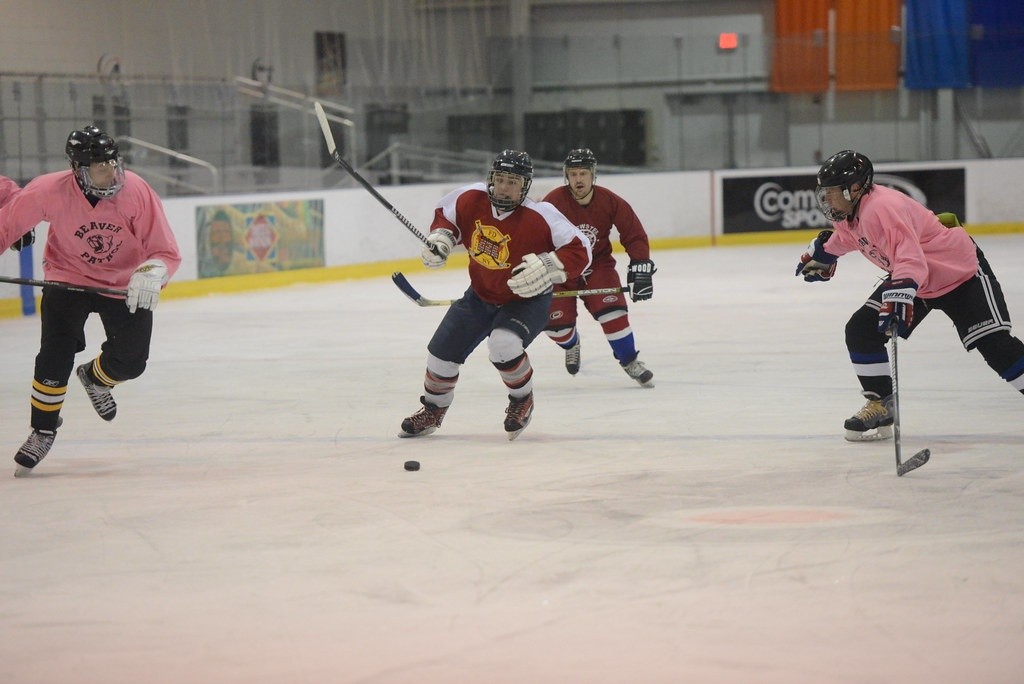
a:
[404,461,420,471]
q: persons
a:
[0,126,181,477]
[401,150,593,441]
[540,148,655,388]
[795,150,1024,442]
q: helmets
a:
[563,148,597,168]
[64,125,125,200]
[817,150,874,221]
[486,149,534,212]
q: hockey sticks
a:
[392,269,631,307]
[890,321,930,477]
[0,274,128,297]
[314,101,440,256]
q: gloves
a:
[875,278,918,337]
[628,259,658,302]
[795,230,838,282]
[125,259,169,313]
[11,227,35,252]
[421,227,456,269]
[507,251,566,298]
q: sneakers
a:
[504,389,534,441]
[844,391,895,441]
[398,396,449,438]
[13,416,63,477]
[565,332,581,374]
[613,351,656,387]
[76,359,117,422]
